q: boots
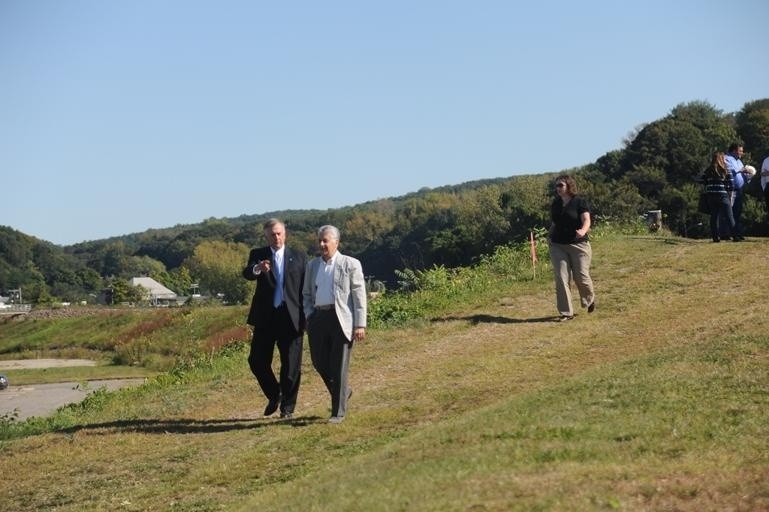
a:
[714,232,744,242]
[329,416,345,424]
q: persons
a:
[548,175,596,322]
[301,225,368,423]
[242,219,307,419]
[760,157,769,202]
[704,142,752,242]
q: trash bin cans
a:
[647,210,662,233]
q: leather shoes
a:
[264,391,279,416]
[588,303,594,312]
[559,316,573,322]
[279,411,291,418]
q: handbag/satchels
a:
[698,193,711,215]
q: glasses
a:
[555,183,565,187]
